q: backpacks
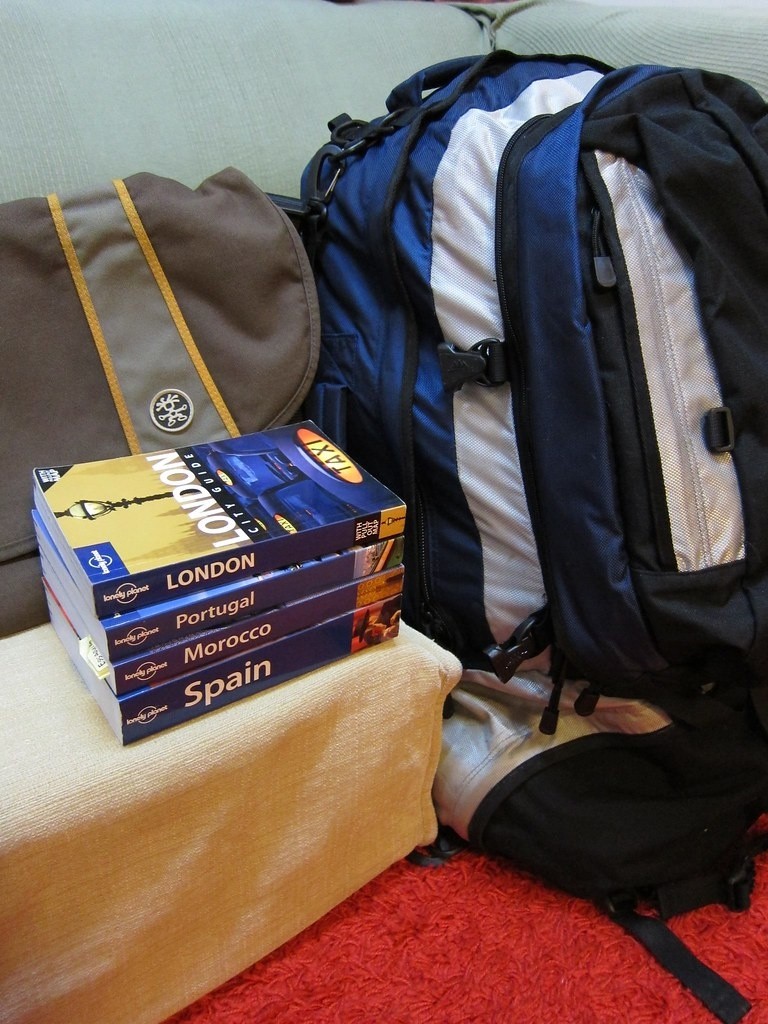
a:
[294,49,768,735]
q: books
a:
[31,420,406,747]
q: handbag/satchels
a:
[0,167,320,639]
